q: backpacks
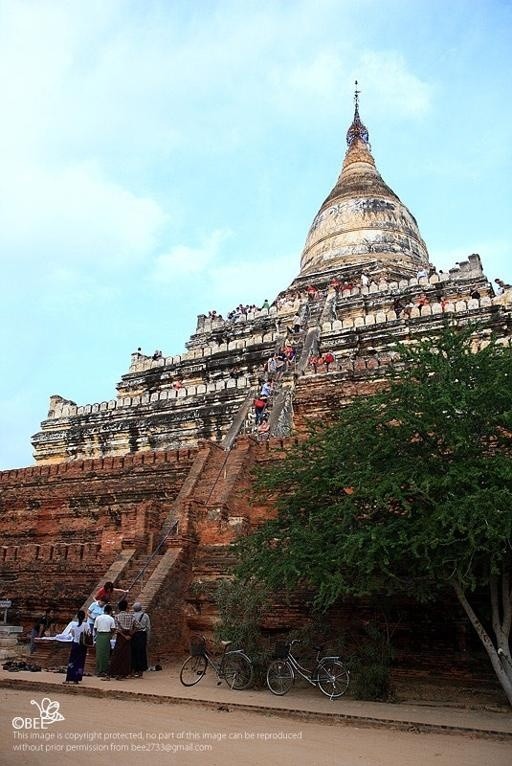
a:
[264,358,273,371]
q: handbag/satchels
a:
[80,624,94,648]
[255,397,265,408]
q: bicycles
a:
[266,639,351,700]
[179,632,254,691]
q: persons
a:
[307,348,335,373]
[151,347,166,361]
[254,334,302,434]
[207,260,511,335]
[172,377,187,388]
[61,582,152,684]
